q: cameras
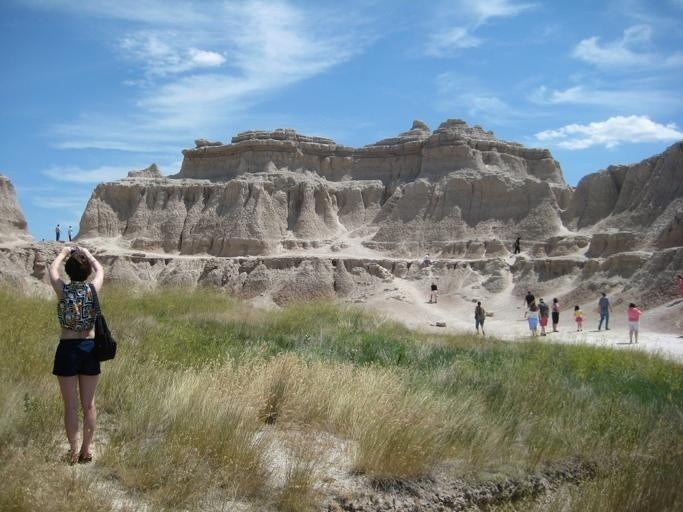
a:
[71,249,76,254]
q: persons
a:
[49,245,105,466]
[627,302,642,344]
[474,301,487,337]
[514,235,522,254]
[421,252,432,267]
[54,223,61,241]
[523,290,561,338]
[676,274,683,300]
[428,281,439,304]
[573,304,584,332]
[598,291,611,331]
[67,225,73,241]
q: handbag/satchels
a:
[87,283,118,363]
